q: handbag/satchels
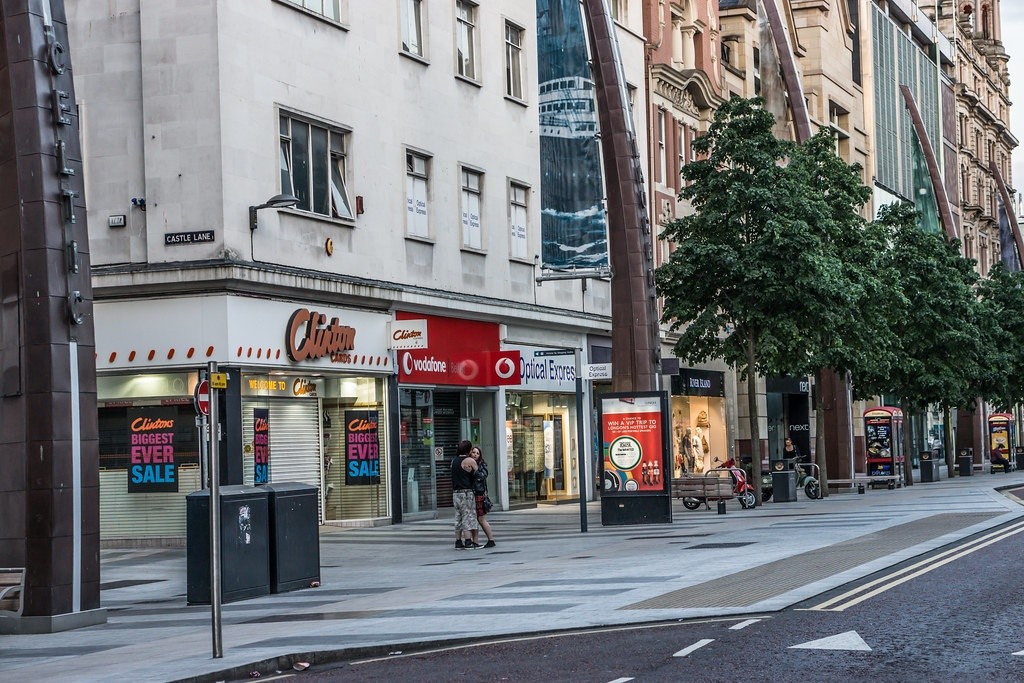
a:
[482,496,491,515]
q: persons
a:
[783,438,801,482]
[673,425,704,477]
[991,444,1013,473]
[450,440,484,549]
[470,447,496,548]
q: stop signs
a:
[195,379,211,417]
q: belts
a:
[453,489,473,493]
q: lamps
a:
[249,193,301,230]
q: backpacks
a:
[701,436,709,453]
[696,411,708,427]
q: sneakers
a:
[465,542,484,550]
[454,541,465,549]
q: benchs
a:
[827,479,870,494]
[671,477,734,511]
[0,567,26,619]
[855,476,901,489]
[990,462,1017,474]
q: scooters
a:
[746,455,820,502]
[681,459,757,510]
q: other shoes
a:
[484,540,495,548]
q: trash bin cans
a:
[958,447,974,476]
[771,459,799,503]
[933,447,941,459]
[1015,446,1024,470]
[920,450,940,483]
